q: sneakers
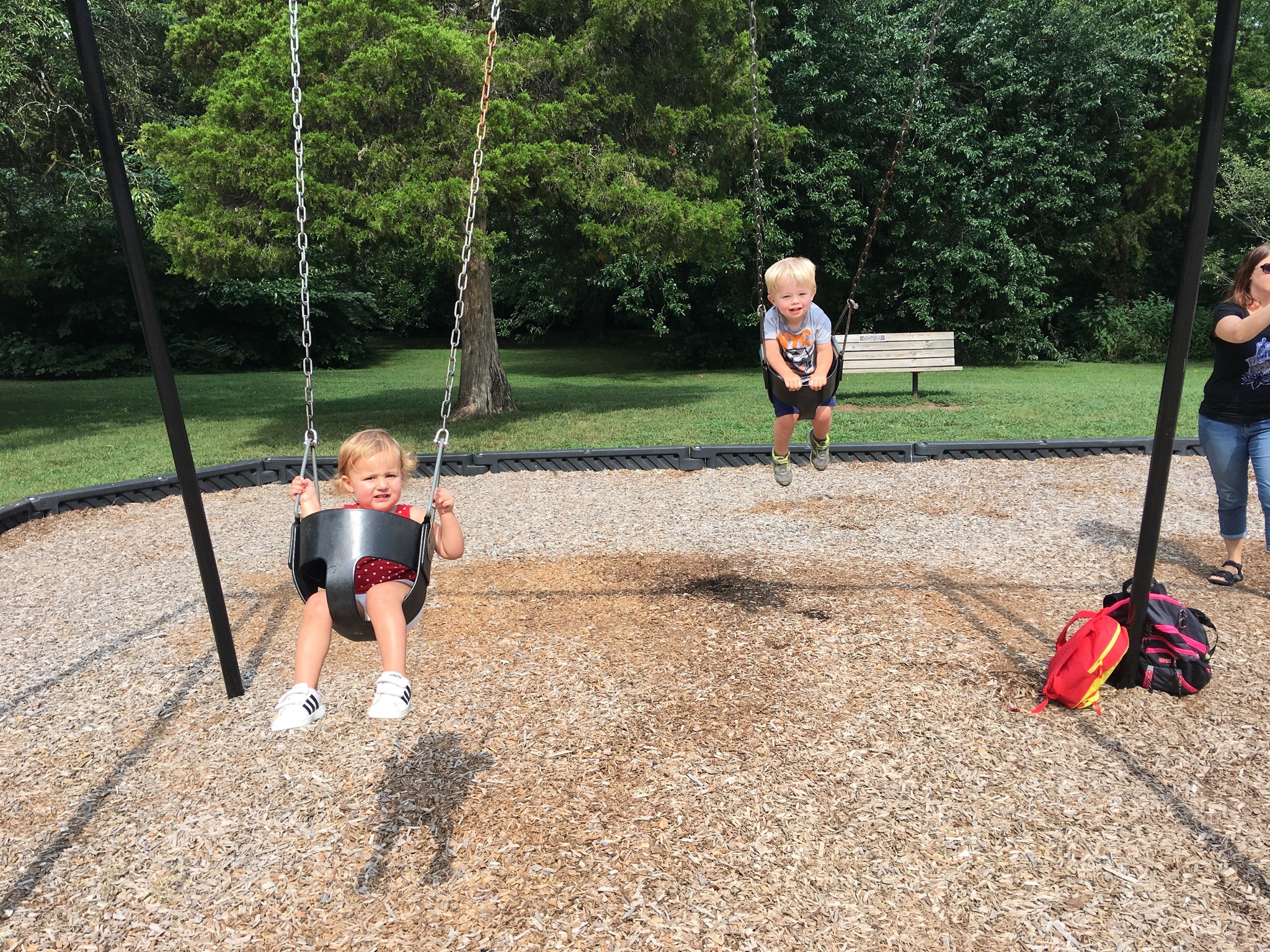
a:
[271,682,326,732]
[808,428,830,471]
[368,671,413,719]
[772,445,794,487]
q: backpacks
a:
[1042,607,1130,714]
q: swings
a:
[749,0,948,419]
[280,0,503,642]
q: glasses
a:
[1254,263,1270,274]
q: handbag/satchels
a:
[1103,576,1219,698]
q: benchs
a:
[832,331,963,400]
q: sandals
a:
[1208,560,1244,586]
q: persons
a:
[759,256,836,487]
[270,428,466,730]
[1199,243,1270,585]
